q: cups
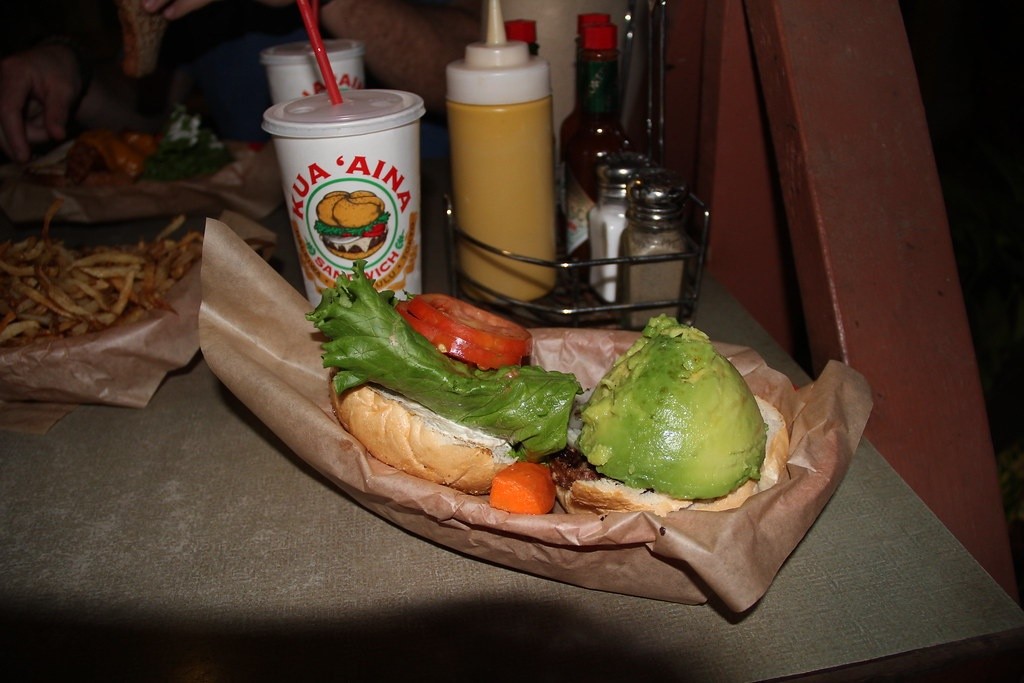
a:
[261,89,427,309]
[258,37,366,104]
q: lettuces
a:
[305,260,584,460]
[136,103,231,182]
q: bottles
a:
[444,0,558,304]
[615,173,687,330]
[564,23,629,277]
[588,154,642,303]
[503,19,540,56]
[558,11,612,161]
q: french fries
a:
[0,196,204,346]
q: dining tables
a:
[0,199,1024,683]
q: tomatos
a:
[396,293,531,370]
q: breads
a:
[328,366,520,494]
[555,397,791,519]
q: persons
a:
[0,0,480,167]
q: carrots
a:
[489,460,557,515]
